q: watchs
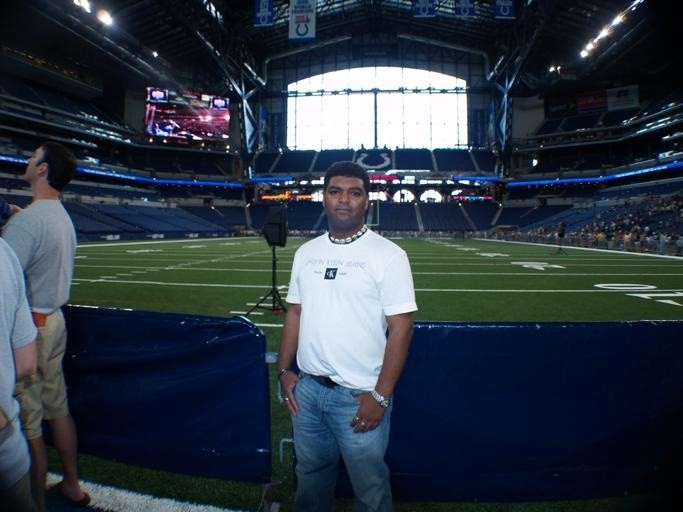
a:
[369,389,391,409]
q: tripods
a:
[244,247,287,317]
[555,237,568,256]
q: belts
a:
[311,375,339,389]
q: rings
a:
[282,398,288,401]
[360,421,365,427]
[353,416,359,424]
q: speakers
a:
[558,229,564,237]
[264,205,287,247]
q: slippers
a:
[48,481,91,506]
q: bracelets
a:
[276,367,294,379]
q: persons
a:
[0,139,90,511]
[237,227,487,240]
[146,86,230,142]
[0,194,23,229]
[0,235,40,511]
[486,193,682,257]
[274,161,418,512]
